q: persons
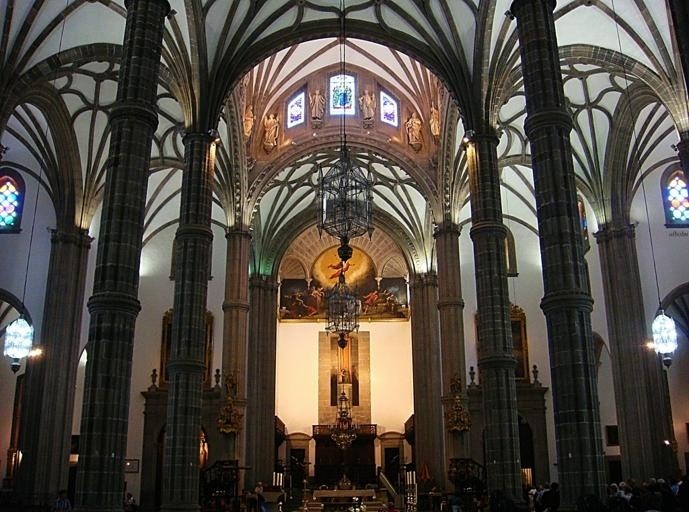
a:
[358,89,376,119]
[126,493,135,505]
[429,104,440,136]
[528,481,560,512]
[405,112,422,143]
[309,90,326,120]
[282,259,408,319]
[263,113,279,145]
[244,105,254,134]
[255,482,264,494]
[339,473,351,490]
[608,478,689,512]
[54,489,71,512]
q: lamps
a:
[315,1,376,423]
[3,0,70,376]
[609,0,680,373]
